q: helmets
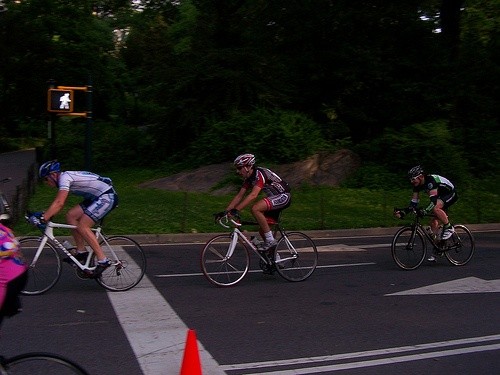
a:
[408,165,423,179]
[39,160,60,179]
[233,153,256,168]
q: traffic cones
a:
[178,326,203,375]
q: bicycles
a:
[0,351,90,375]
[13,210,147,296]
[201,209,319,287]
[390,207,476,270]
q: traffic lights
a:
[47,88,74,113]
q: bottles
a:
[437,225,443,240]
[250,235,261,248]
[426,227,436,240]
[62,240,76,256]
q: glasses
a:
[410,174,423,182]
[237,167,243,170]
[44,176,49,181]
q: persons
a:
[0,224,30,322]
[29,159,117,279]
[215,153,291,274]
[394,165,457,261]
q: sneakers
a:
[91,258,113,278]
[62,251,89,262]
[277,263,285,269]
[258,239,277,251]
[427,253,444,262]
[442,225,454,240]
